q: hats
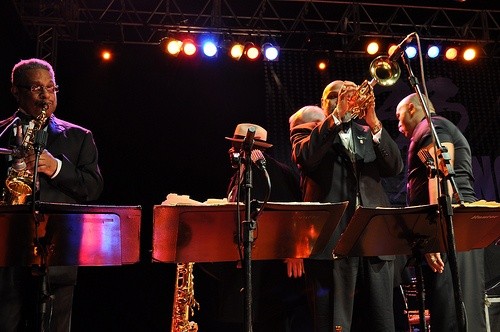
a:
[224,123,273,148]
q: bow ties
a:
[341,119,352,133]
[16,110,50,130]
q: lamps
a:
[158,36,280,62]
[355,34,478,61]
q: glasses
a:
[16,83,59,94]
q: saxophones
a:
[171,263,198,332]
[4,106,50,205]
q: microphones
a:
[418,148,437,178]
[389,32,415,62]
[0,116,21,138]
[31,130,49,222]
[238,126,256,161]
[250,148,271,220]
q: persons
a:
[291,79,403,332]
[396,92,486,332]
[283,104,328,279]
[225,123,274,205]
[0,56,104,332]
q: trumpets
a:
[339,56,401,123]
